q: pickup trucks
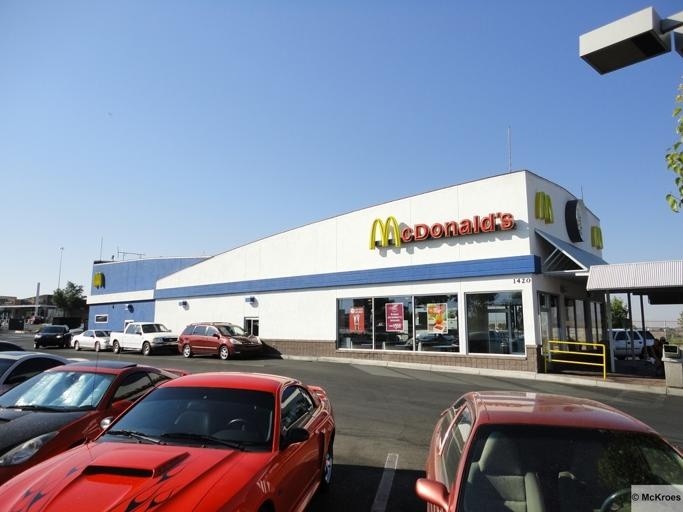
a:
[109,322,179,356]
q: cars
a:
[353,322,521,351]
[178,322,264,360]
[71,329,112,352]
[416,390,683,512]
[33,323,72,348]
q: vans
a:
[607,329,655,360]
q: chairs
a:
[462,429,547,512]
[175,399,215,439]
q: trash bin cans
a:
[661,344,683,387]
